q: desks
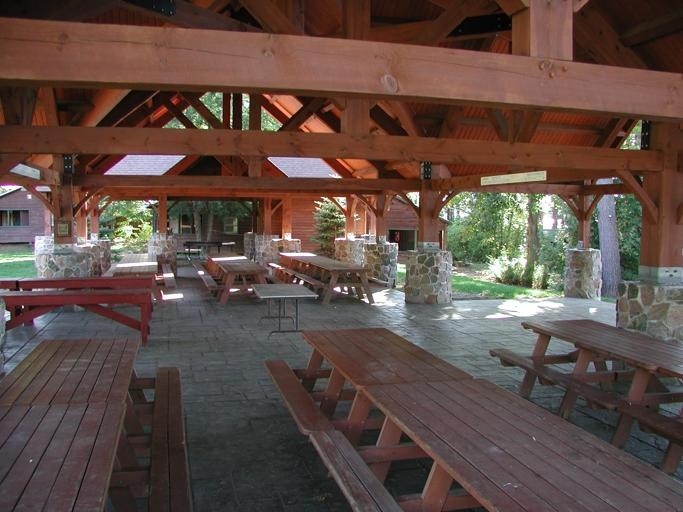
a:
[277,252,375,306]
[208,255,270,305]
[0,287,154,343]
[300,328,471,425]
[0,405,150,511]
[251,284,319,339]
[518,320,683,448]
[17,274,155,313]
[0,277,21,292]
[113,254,166,305]
[0,335,149,458]
[348,378,683,512]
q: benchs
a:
[152,366,193,512]
[160,263,177,290]
[268,262,296,283]
[612,404,683,475]
[293,272,325,294]
[304,431,403,511]
[262,359,335,439]
[193,262,217,293]
[489,348,621,431]
